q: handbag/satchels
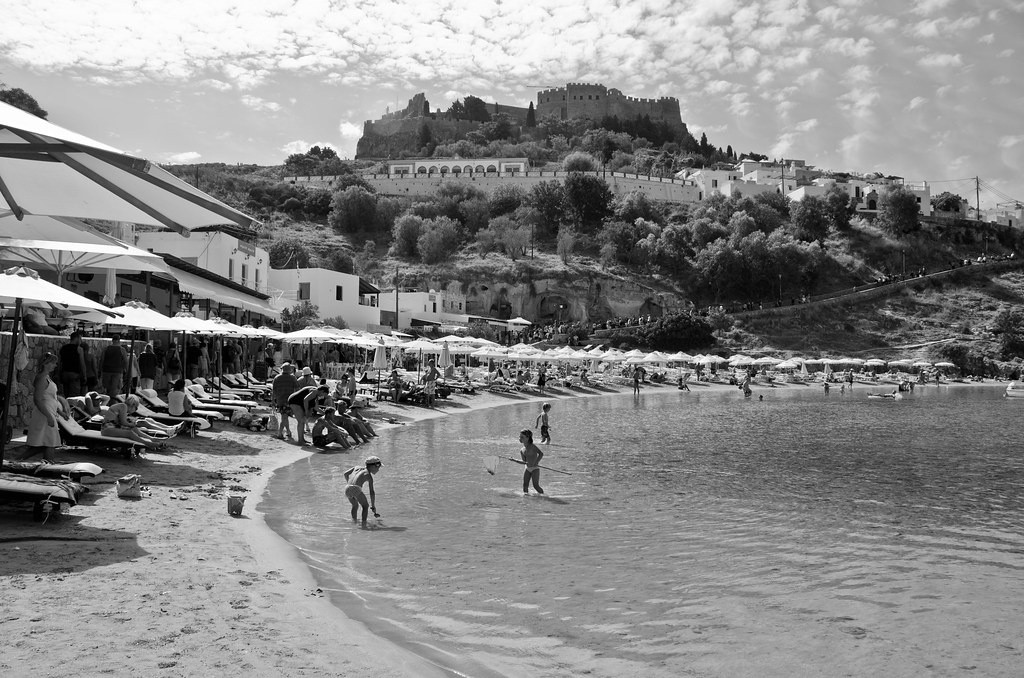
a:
[167,357,180,375]
[14,330,30,370]
[116,473,142,497]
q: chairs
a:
[0,361,640,522]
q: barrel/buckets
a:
[228,494,245,515]
[116,475,140,497]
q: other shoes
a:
[285,435,293,440]
[298,442,310,446]
[277,435,285,439]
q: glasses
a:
[133,403,138,411]
[44,352,51,361]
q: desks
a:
[198,398,220,404]
[231,384,247,389]
[86,418,103,431]
[361,388,376,396]
[252,382,265,385]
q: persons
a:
[688,299,764,318]
[534,403,552,444]
[822,366,941,396]
[777,292,810,305]
[963,250,1017,266]
[388,358,767,409]
[876,265,927,286]
[343,455,382,522]
[60,332,378,450]
[511,429,544,493]
[505,313,651,347]
[24,352,68,461]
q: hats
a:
[365,456,380,463]
[521,429,532,437]
[281,362,294,372]
[301,367,313,375]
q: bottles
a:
[140,491,151,497]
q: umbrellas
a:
[286,321,726,382]
[507,317,531,325]
[0,264,283,465]
[730,353,956,378]
[0,100,264,285]
